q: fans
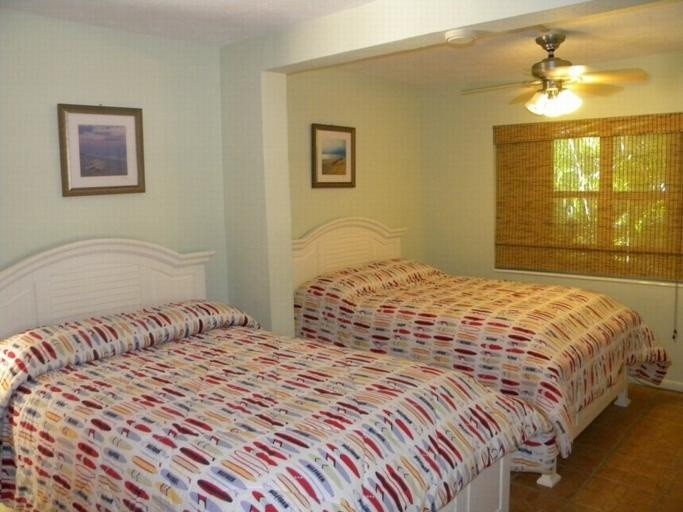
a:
[458,33,650,104]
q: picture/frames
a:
[56,101,147,197]
[311,120,356,188]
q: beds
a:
[292,216,632,489]
[0,238,514,512]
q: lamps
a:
[522,83,581,118]
